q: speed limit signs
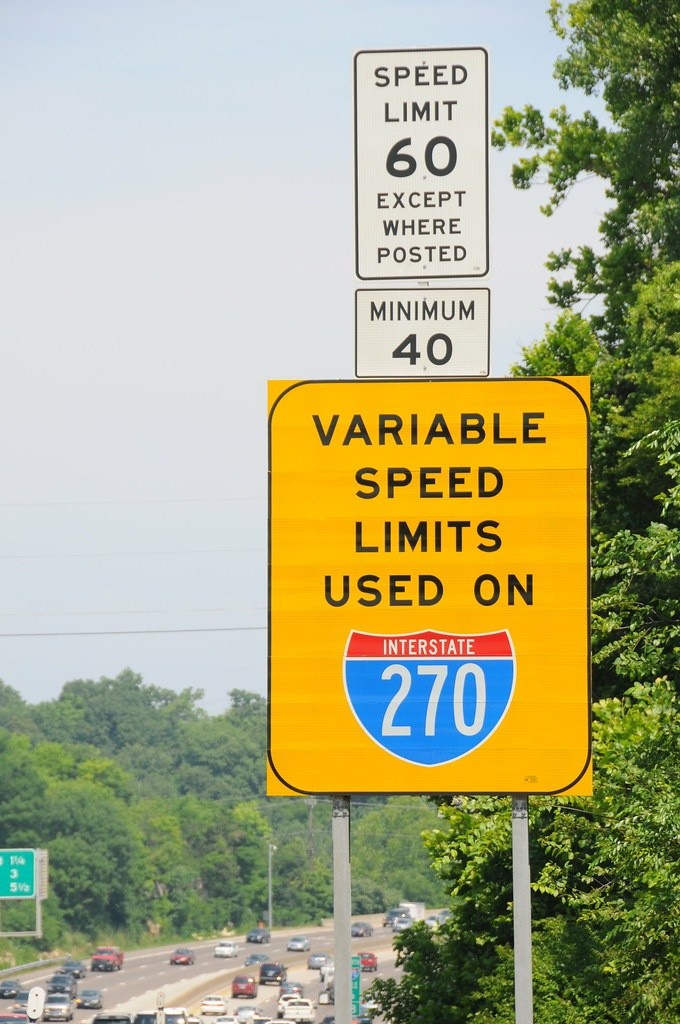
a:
[353,47,491,279]
[354,287,489,378]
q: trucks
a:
[89,946,124,972]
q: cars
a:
[351,921,373,937]
[0,960,318,1024]
[167,948,195,965]
[318,959,385,1024]
[0,978,22,999]
[213,940,239,958]
[306,952,328,969]
[245,952,270,966]
[382,906,451,932]
[286,936,311,952]
[246,927,271,943]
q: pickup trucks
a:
[357,952,378,972]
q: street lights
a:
[268,844,277,927]
